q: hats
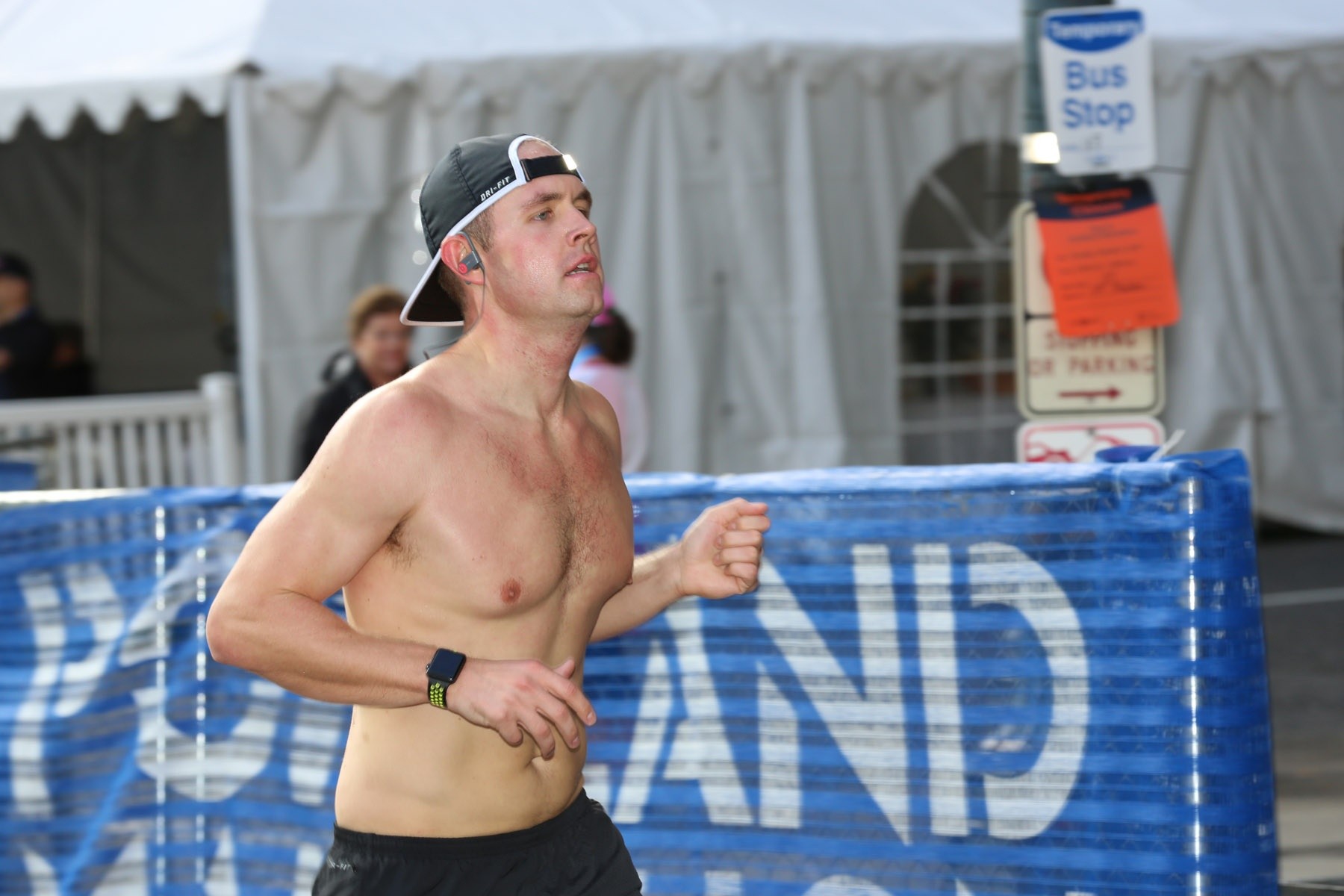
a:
[398,131,586,328]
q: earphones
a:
[454,231,484,274]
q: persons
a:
[294,285,416,482]
[205,133,771,896]
[567,290,650,472]
[0,249,98,399]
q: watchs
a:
[426,647,466,709]
